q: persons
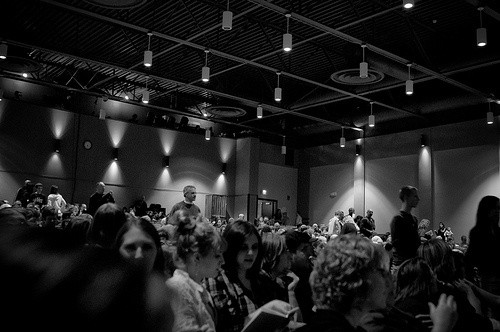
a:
[390,184,421,261]
[0,181,500,331]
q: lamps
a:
[340,103,427,155]
[55,127,228,174]
[487,99,493,125]
[222,0,233,31]
[405,64,414,95]
[143,76,150,103]
[403,0,415,9]
[283,12,292,52]
[144,34,153,68]
[202,50,210,82]
[99,97,106,120]
[274,73,281,101]
[477,7,487,46]
[281,136,286,154]
[257,104,264,118]
[360,44,368,77]
